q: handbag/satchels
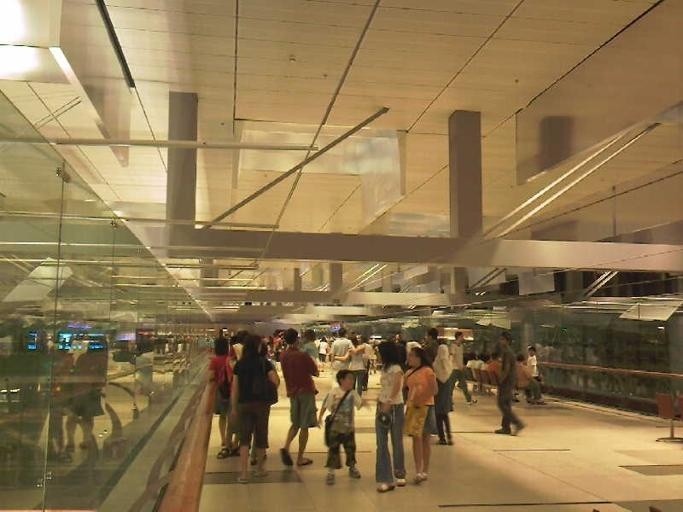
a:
[261,379,280,405]
[218,380,232,398]
[378,409,395,427]
[323,416,334,446]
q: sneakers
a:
[326,471,336,486]
[437,439,453,446]
[348,468,361,478]
[494,423,526,437]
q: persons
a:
[230,334,280,482]
[449,331,478,405]
[494,331,524,434]
[0,311,200,474]
[538,340,673,402]
[318,369,369,485]
[210,328,454,459]
[404,347,438,484]
[279,328,319,466]
[467,346,538,404]
[376,341,406,493]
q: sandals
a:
[235,470,270,483]
[413,471,427,486]
[217,448,243,459]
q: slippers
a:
[378,479,406,492]
[281,445,315,466]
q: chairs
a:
[654,391,683,442]
[459,365,520,403]
